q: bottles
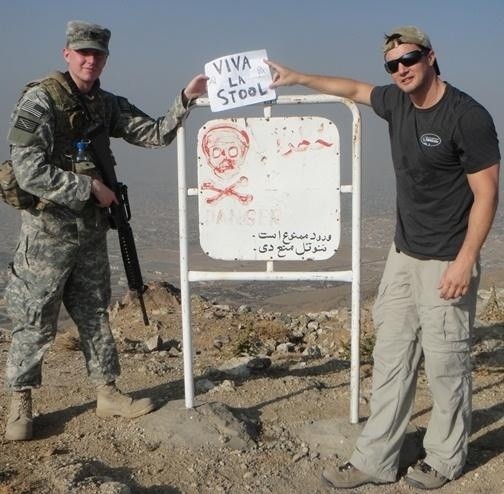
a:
[73,142,97,176]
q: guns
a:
[74,122,150,328]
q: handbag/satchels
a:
[0,159,37,210]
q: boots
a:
[96,381,156,419]
[5,390,33,440]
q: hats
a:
[382,26,440,75]
[65,21,111,54]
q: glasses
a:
[384,48,427,74]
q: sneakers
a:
[321,462,389,488]
[406,462,448,490]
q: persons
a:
[262,25,502,490]
[2,18,208,444]
